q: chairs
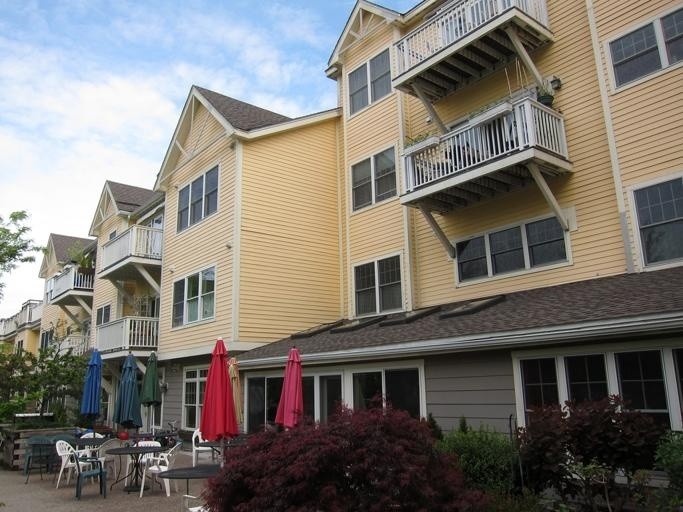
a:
[22,428,221,512]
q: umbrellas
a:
[111,350,143,430]
[199,337,239,468]
[138,351,162,432]
[80,348,101,420]
[274,344,305,430]
[227,357,244,426]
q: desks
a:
[194,441,246,455]
